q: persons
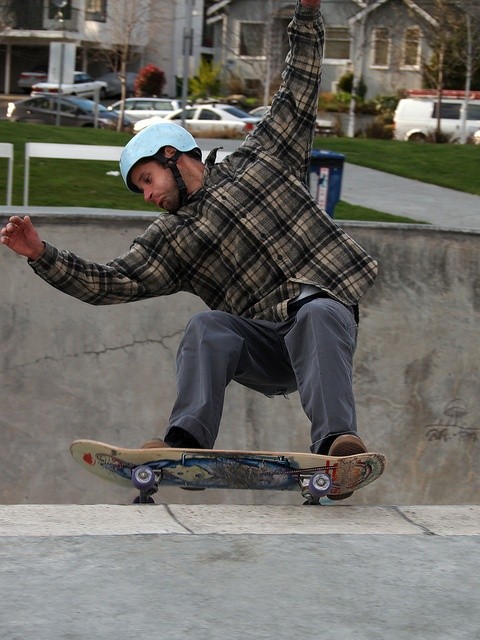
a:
[0,0,378,501]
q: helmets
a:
[119,122,202,194]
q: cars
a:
[17,68,47,93]
[30,71,112,103]
[248,105,340,138]
[133,101,264,142]
[6,92,134,137]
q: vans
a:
[391,96,480,144]
[106,94,184,127]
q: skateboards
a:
[70,438,389,505]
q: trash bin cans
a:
[306,149,345,219]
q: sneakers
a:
[327,434,368,500]
[140,438,207,490]
